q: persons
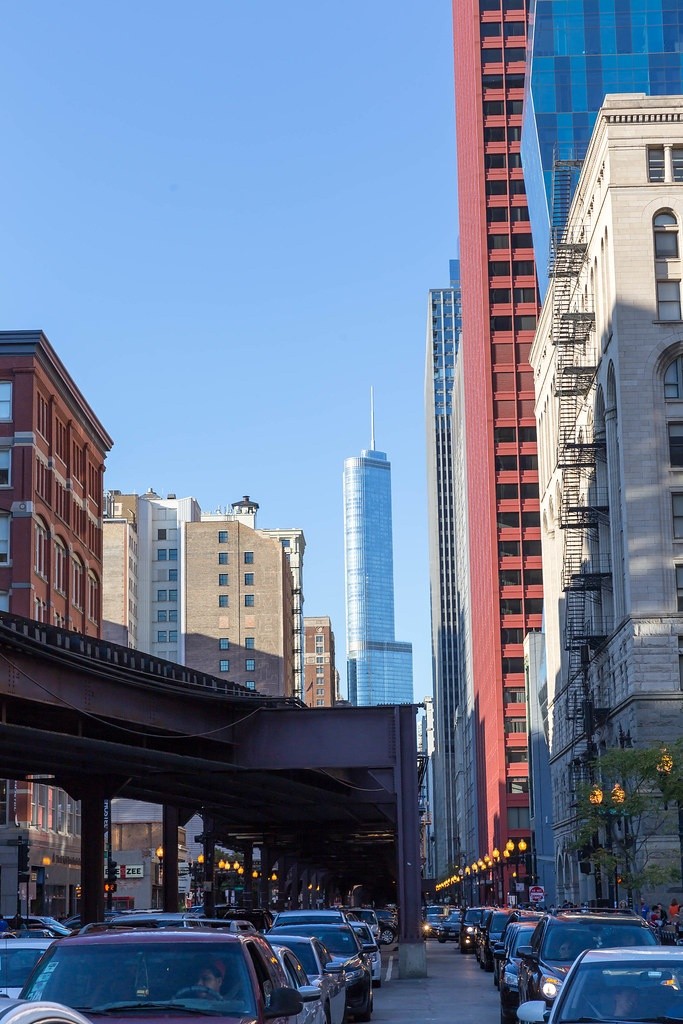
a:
[618,898,680,945]
[614,987,639,1017]
[9,913,24,930]
[58,912,68,922]
[194,960,226,1001]
[0,914,10,938]
[492,900,589,917]
[559,940,572,957]
[622,933,636,946]
[455,908,466,949]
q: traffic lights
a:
[104,861,118,892]
[18,846,30,883]
[75,886,81,900]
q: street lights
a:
[589,783,626,909]
[656,748,683,873]
[156,846,244,906]
[434,838,527,909]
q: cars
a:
[0,910,258,1024]
[17,926,303,1024]
[263,908,399,1024]
[422,907,683,1024]
